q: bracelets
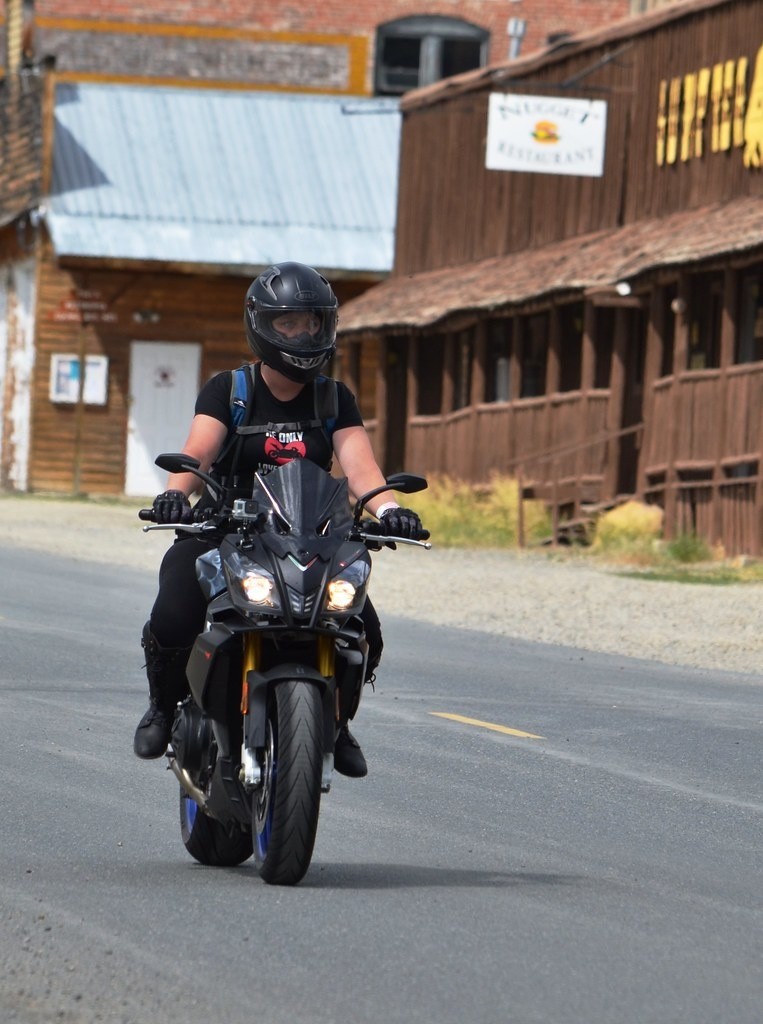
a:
[376,502,400,519]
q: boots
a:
[133,619,194,759]
[334,653,383,777]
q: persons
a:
[133,262,423,778]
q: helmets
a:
[243,261,340,384]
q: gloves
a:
[153,489,192,524]
[378,507,430,551]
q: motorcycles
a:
[137,451,435,887]
